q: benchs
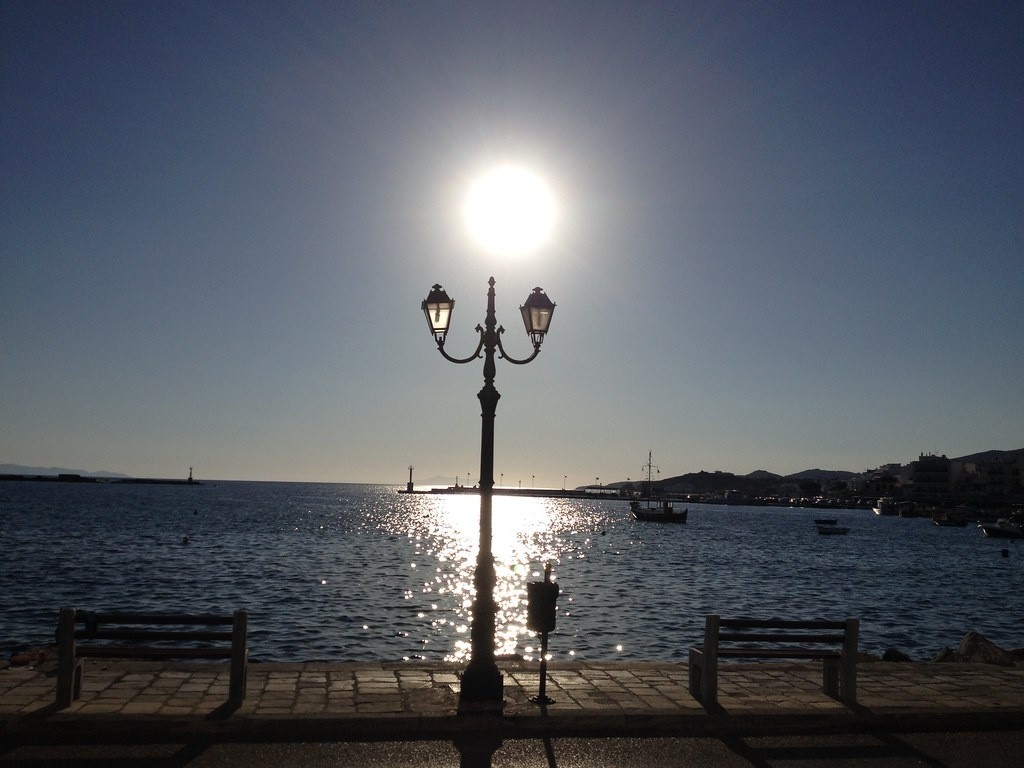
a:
[689,615,859,704]
[54,605,253,707]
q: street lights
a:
[564,475,567,489]
[596,477,599,485]
[501,473,503,486]
[532,475,535,488]
[519,481,521,488]
[420,275,559,709]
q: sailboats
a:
[630,449,688,525]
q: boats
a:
[929,498,971,528]
[896,501,926,517]
[814,519,837,525]
[816,525,851,535]
[872,497,898,516]
[976,504,1024,540]
